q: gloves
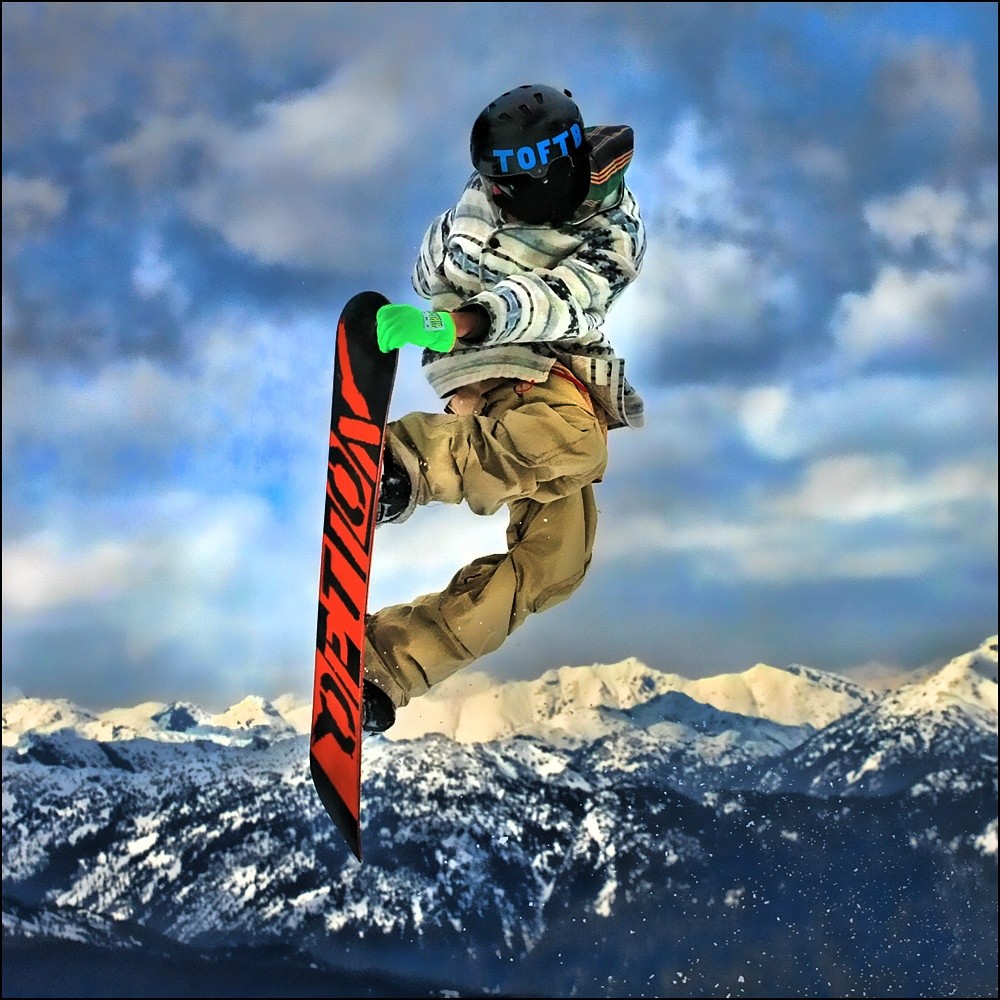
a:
[376,304,457,353]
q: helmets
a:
[468,84,591,222]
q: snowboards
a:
[308,293,401,856]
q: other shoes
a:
[362,678,396,732]
[376,445,410,522]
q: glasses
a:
[478,170,544,197]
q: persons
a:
[360,83,644,733]
